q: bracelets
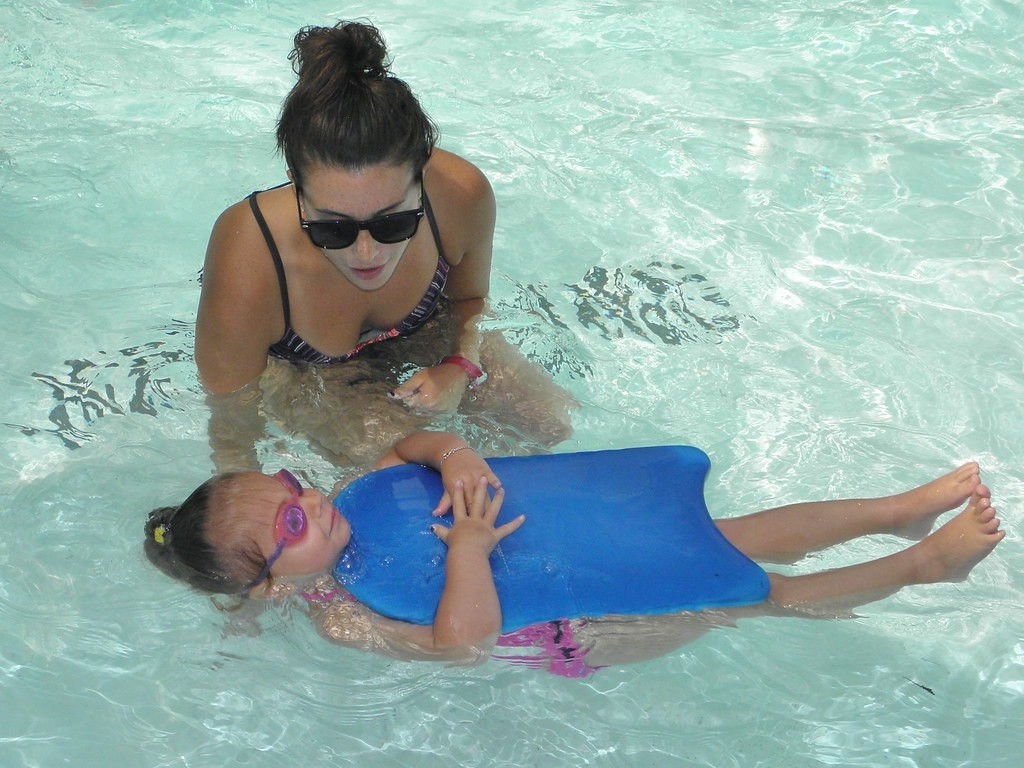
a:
[442,447,477,473]
[434,354,489,392]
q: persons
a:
[195,20,581,462]
[143,425,1007,666]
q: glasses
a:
[238,468,308,594]
[290,165,425,249]
